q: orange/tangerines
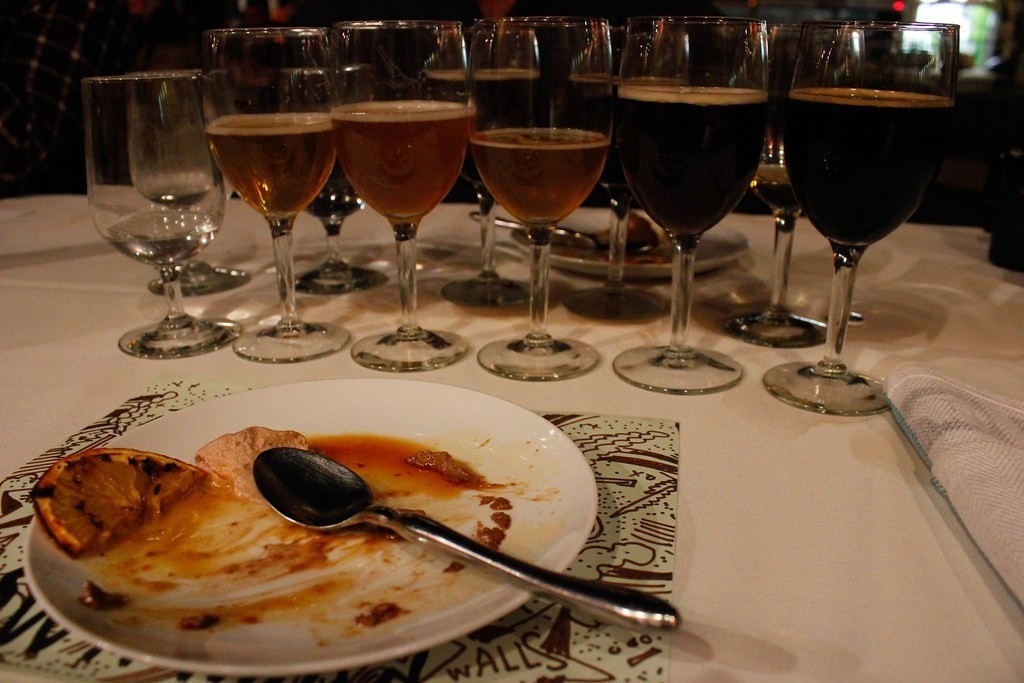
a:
[31,448,206,560]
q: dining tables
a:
[0,182,1023,679]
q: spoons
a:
[252,445,684,633]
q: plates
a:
[22,376,599,674]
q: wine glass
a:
[81,15,960,415]
